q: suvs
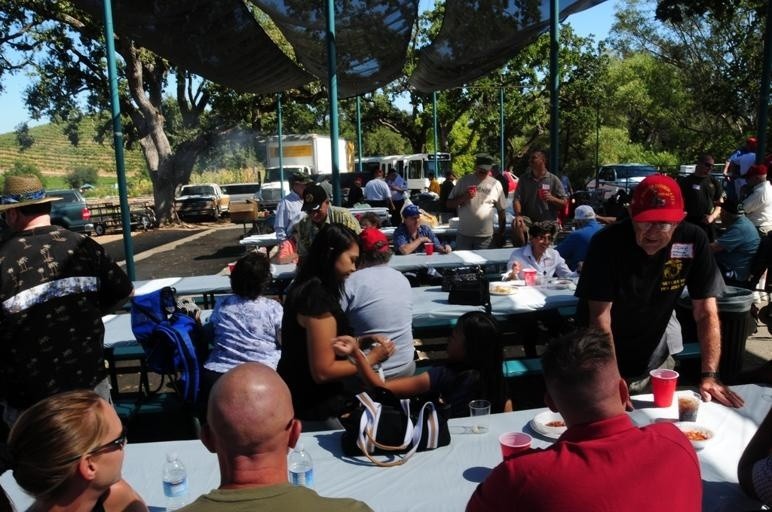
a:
[45,187,93,236]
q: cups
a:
[425,243,433,255]
[677,391,702,423]
[469,399,491,434]
[649,368,680,407]
[265,212,270,216]
[499,433,532,462]
[539,189,548,200]
[468,185,476,195]
[521,269,537,287]
[228,263,236,272]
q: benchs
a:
[503,358,543,378]
[106,344,153,401]
[675,341,701,360]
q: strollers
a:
[123,285,239,428]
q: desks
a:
[218,267,233,278]
[1,384,772,512]
[378,227,397,240]
[431,223,459,238]
[269,261,299,295]
[101,313,149,350]
[391,248,516,279]
[410,274,578,351]
[129,274,234,311]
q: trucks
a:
[256,132,356,208]
[86,200,156,236]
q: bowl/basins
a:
[672,421,716,449]
[549,278,573,289]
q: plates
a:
[448,217,460,229]
[489,284,519,296]
[530,410,566,441]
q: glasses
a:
[699,160,715,168]
[54,431,130,470]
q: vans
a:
[218,183,260,202]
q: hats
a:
[474,153,495,173]
[402,205,422,216]
[739,164,769,178]
[287,171,315,192]
[713,200,746,215]
[0,171,64,211]
[627,175,687,226]
[574,204,597,222]
[745,136,757,145]
[360,226,390,254]
[301,182,327,213]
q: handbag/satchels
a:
[440,267,484,292]
[339,384,454,466]
[447,281,491,306]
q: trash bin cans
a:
[676,285,754,386]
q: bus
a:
[353,151,448,201]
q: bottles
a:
[287,440,318,497]
[162,450,190,512]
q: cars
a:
[172,183,231,220]
[81,184,94,191]
[569,159,730,202]
